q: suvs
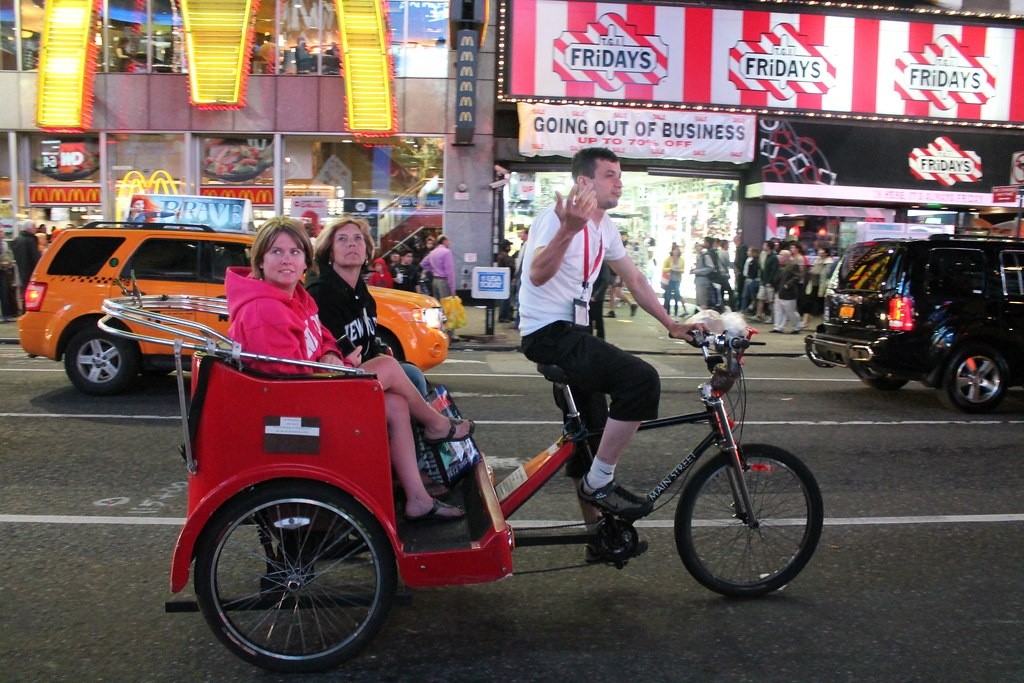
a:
[17,227,449,393]
[805,234,1024,409]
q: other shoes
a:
[631,304,637,316]
[3,316,16,321]
[602,311,615,318]
[749,315,762,322]
[765,318,774,323]
[790,331,800,334]
[801,322,808,328]
[770,330,783,333]
[499,316,517,323]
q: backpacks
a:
[702,247,720,279]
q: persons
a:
[520,147,710,565]
[0,220,75,323]
[589,229,843,341]
[115,36,130,72]
[225,215,475,525]
[367,228,528,342]
[252,31,342,74]
[305,218,428,398]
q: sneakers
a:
[583,540,648,563]
[577,472,654,520]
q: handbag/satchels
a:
[440,295,468,328]
[660,273,671,286]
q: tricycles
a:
[97,281,830,665]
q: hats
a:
[500,240,513,246]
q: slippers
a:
[403,498,464,523]
[425,477,450,496]
[422,416,475,445]
